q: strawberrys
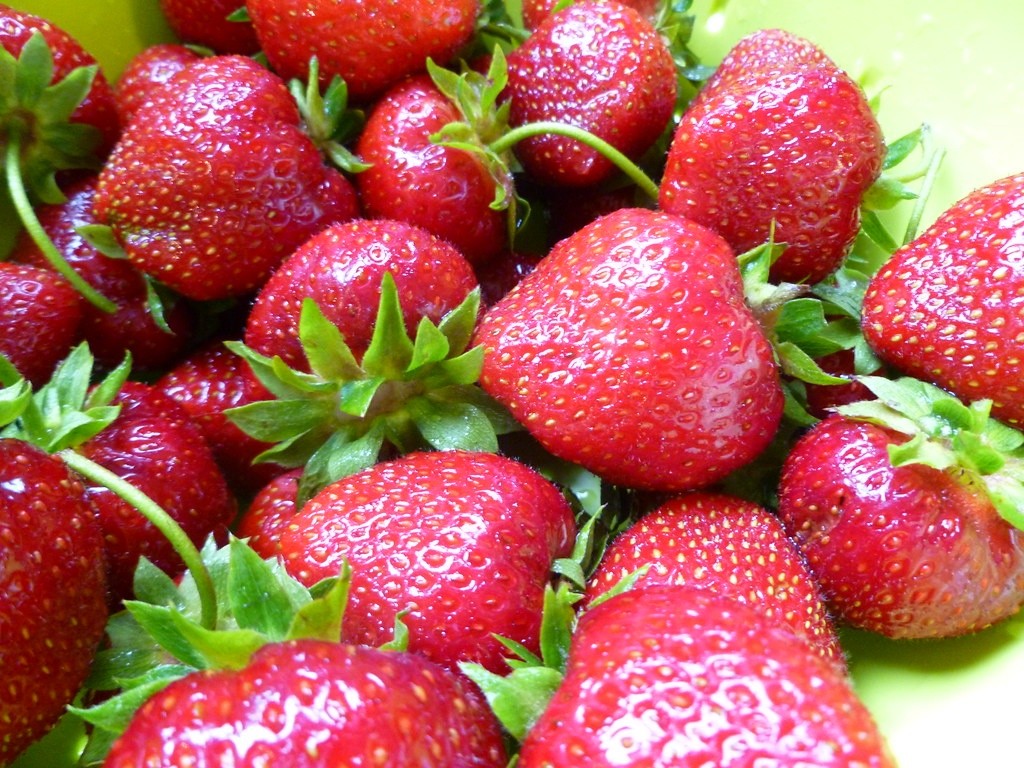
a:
[0,0,1024,768]
